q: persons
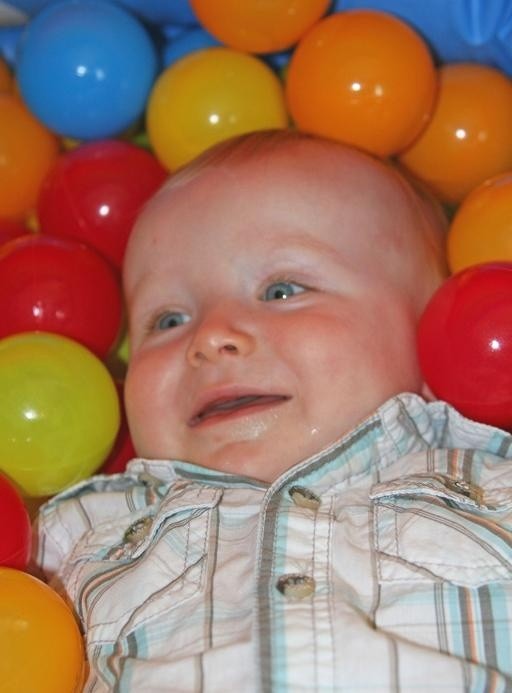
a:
[28,130,512,693]
